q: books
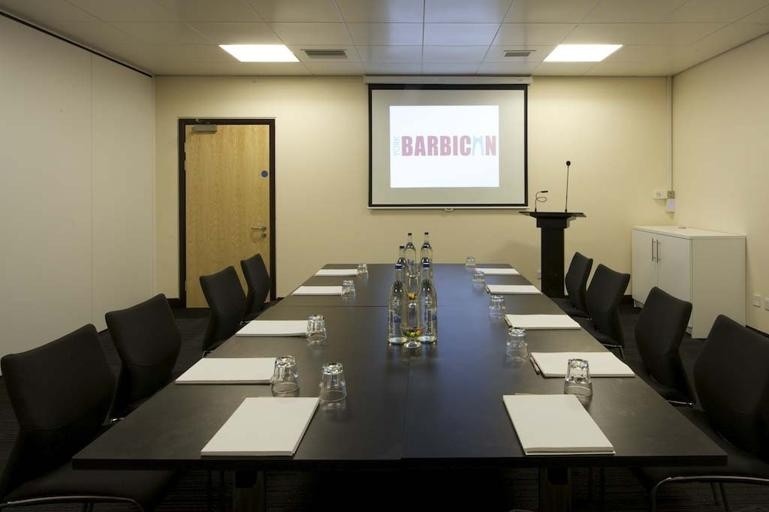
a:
[529,351,636,378]
[290,285,342,296]
[312,268,360,277]
[200,396,319,458]
[474,267,521,277]
[486,284,542,296]
[174,357,278,384]
[234,319,311,336]
[502,313,581,330]
[500,393,616,455]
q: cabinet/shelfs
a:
[629,225,747,339]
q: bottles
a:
[405,233,416,278]
[408,259,419,300]
[397,246,407,291]
[405,293,421,350]
[420,279,438,344]
[388,263,406,346]
[418,258,438,317]
[421,232,434,277]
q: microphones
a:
[563,159,572,211]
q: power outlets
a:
[763,298,768,308]
[750,294,761,307]
[653,191,667,199]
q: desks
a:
[71,262,730,510]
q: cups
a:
[563,359,593,397]
[271,352,300,392]
[341,280,355,302]
[504,327,529,358]
[357,264,368,280]
[464,256,476,269]
[472,272,485,291]
[488,295,506,319]
[319,360,348,403]
[306,313,327,346]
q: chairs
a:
[623,284,696,407]
[200,266,248,356]
[105,294,184,417]
[651,315,769,511]
[0,322,174,500]
[567,264,631,362]
[550,252,593,320]
[241,253,280,317]
